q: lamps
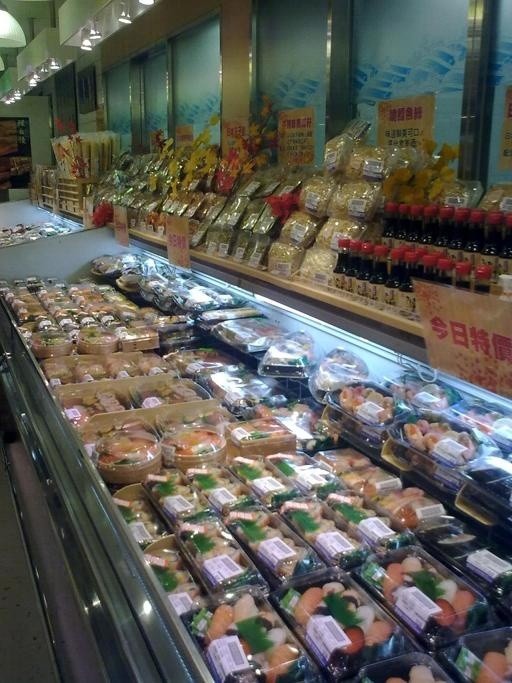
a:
[1,2,154,106]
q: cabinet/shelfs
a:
[26,178,92,221]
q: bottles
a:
[331,199,512,325]
